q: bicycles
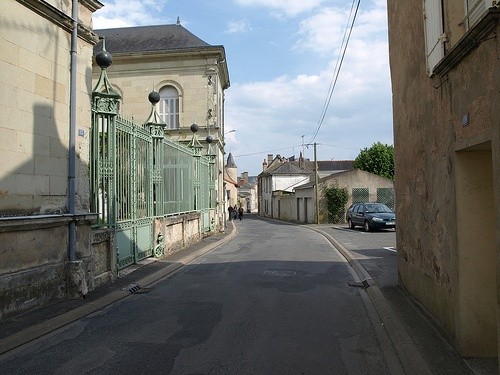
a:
[239,211,243,220]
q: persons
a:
[228,205,244,221]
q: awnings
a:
[224,178,240,188]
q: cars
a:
[346,203,396,232]
[229,206,238,220]
